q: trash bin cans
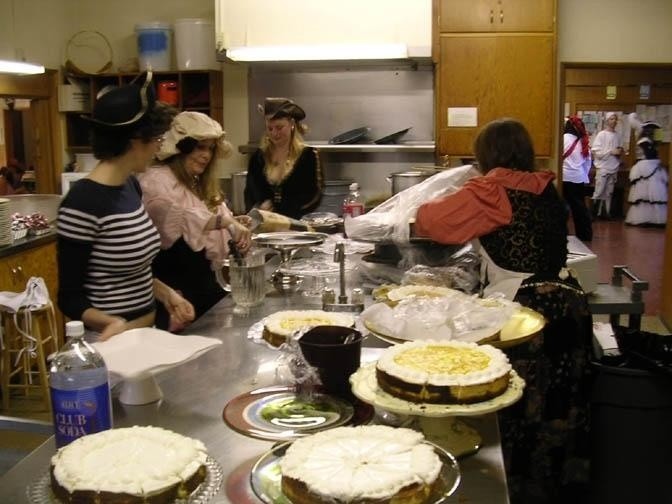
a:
[569,325,672,504]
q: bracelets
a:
[216,210,222,229]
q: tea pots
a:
[212,246,272,310]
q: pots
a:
[386,171,427,198]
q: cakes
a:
[262,309,356,349]
[280,425,447,504]
[375,337,515,406]
[52,423,208,503]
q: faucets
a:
[333,242,348,303]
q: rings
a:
[172,304,181,313]
[243,242,247,245]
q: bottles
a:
[343,183,367,240]
[47,321,110,453]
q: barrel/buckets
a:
[133,20,174,72]
[172,17,222,71]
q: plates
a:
[1,196,12,247]
[365,304,500,346]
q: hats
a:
[257,97,306,121]
[76,70,155,132]
[155,112,232,163]
[643,121,667,131]
[607,111,618,120]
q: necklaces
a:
[270,153,290,171]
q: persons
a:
[140,105,251,337]
[244,96,325,221]
[626,120,669,227]
[561,117,592,245]
[413,115,592,492]
[591,111,626,220]
[57,72,196,344]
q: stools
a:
[0,295,60,412]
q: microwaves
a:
[60,173,90,198]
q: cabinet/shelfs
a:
[431,0,563,191]
[64,70,224,150]
